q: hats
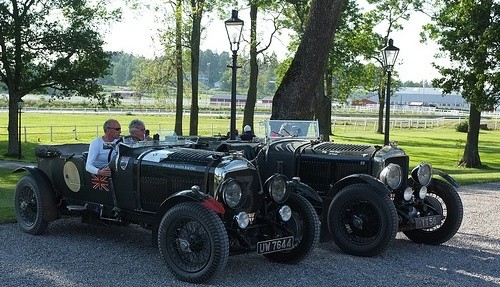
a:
[244,125,251,132]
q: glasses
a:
[108,128,121,131]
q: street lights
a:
[224,9,246,134]
[380,38,399,142]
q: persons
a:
[239,125,256,140]
[279,123,292,136]
[85,119,122,177]
[129,120,146,141]
[227,130,241,140]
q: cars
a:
[13,130,320,283]
[162,118,463,256]
